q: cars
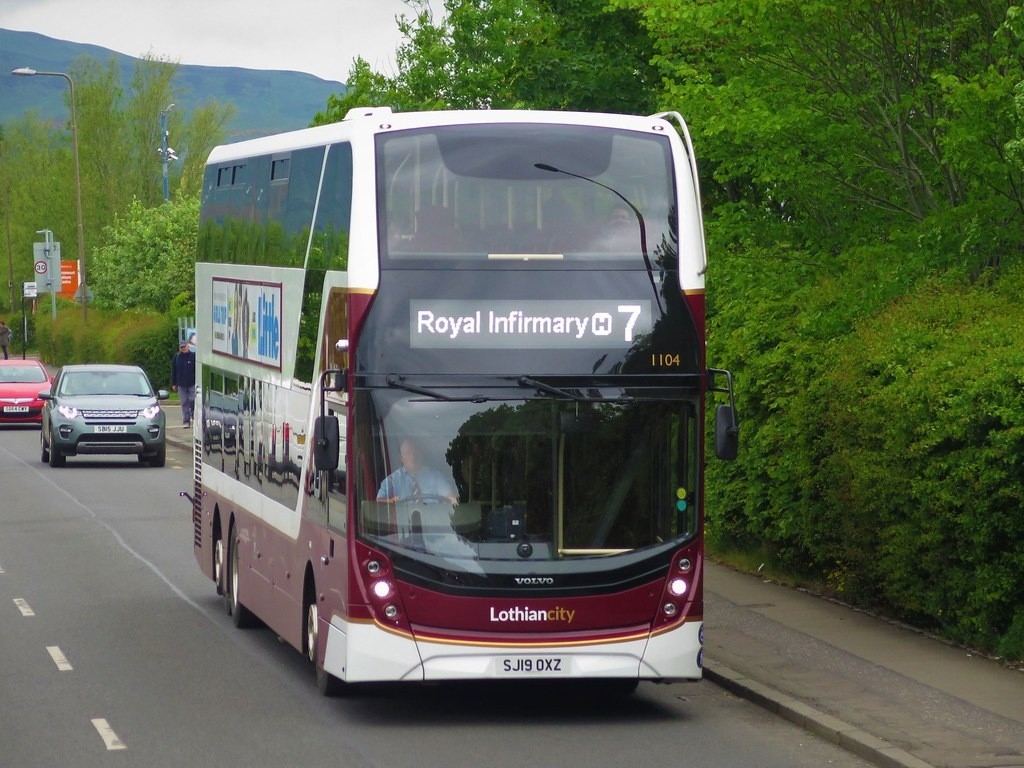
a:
[0,359,58,424]
[38,364,171,468]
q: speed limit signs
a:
[34,260,47,274]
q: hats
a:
[179,340,188,347]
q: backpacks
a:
[4,327,13,340]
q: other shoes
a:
[183,424,190,428]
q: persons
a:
[588,202,634,253]
[172,341,197,428]
[376,435,460,505]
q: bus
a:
[189,107,741,698]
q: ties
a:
[405,472,422,504]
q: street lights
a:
[10,67,88,325]
